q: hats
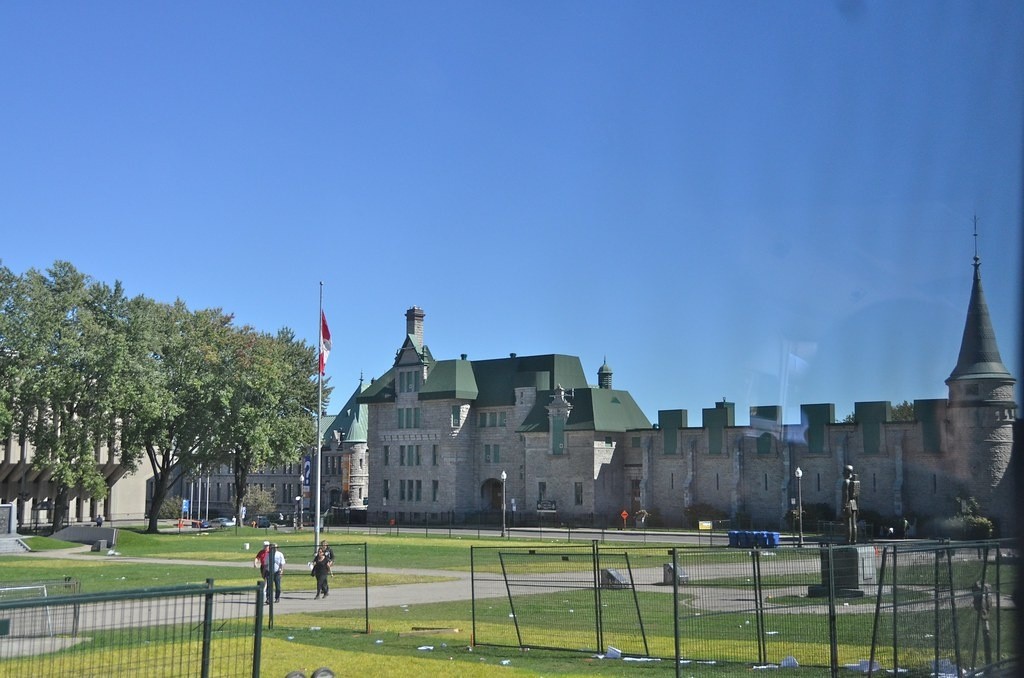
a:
[263,541,270,546]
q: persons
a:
[96,515,103,527]
[254,541,270,592]
[313,540,335,596]
[312,546,332,600]
[839,465,859,544]
[263,543,285,604]
[902,517,909,539]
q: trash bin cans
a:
[727,530,781,548]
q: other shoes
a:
[314,594,320,600]
[266,599,270,604]
[275,597,280,603]
[322,593,329,599]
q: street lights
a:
[500,471,507,537]
[791,496,796,536]
[794,466,804,547]
[299,474,305,529]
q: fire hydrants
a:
[251,521,256,528]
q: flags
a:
[319,309,332,375]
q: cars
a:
[192,519,209,528]
[247,517,270,527]
[211,518,235,527]
